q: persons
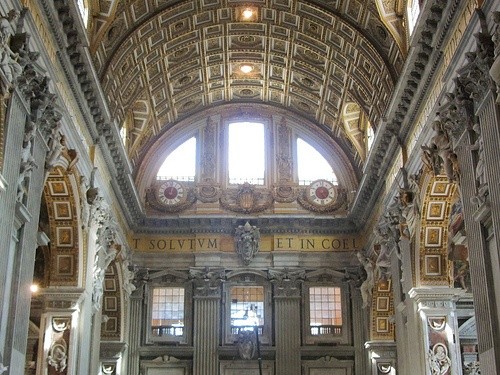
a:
[1,6,500,375]
